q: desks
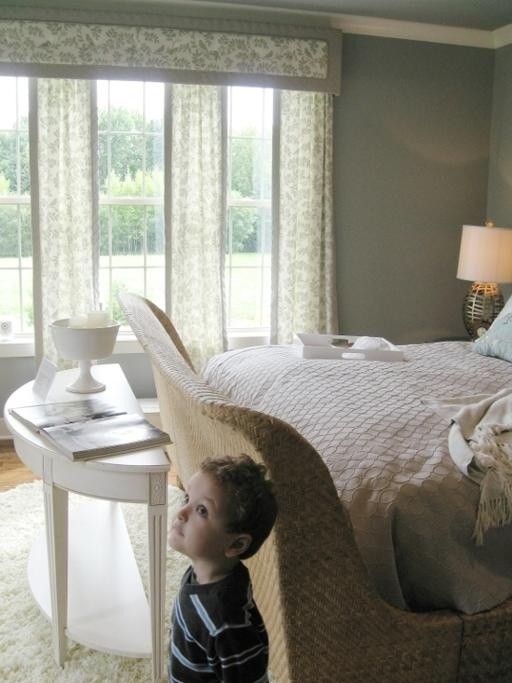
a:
[3,364,170,682]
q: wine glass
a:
[46,314,121,395]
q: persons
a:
[162,451,277,682]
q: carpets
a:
[4,479,191,681]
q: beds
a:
[118,293,510,683]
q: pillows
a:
[470,293,510,362]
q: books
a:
[6,398,172,462]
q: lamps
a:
[453,220,510,341]
